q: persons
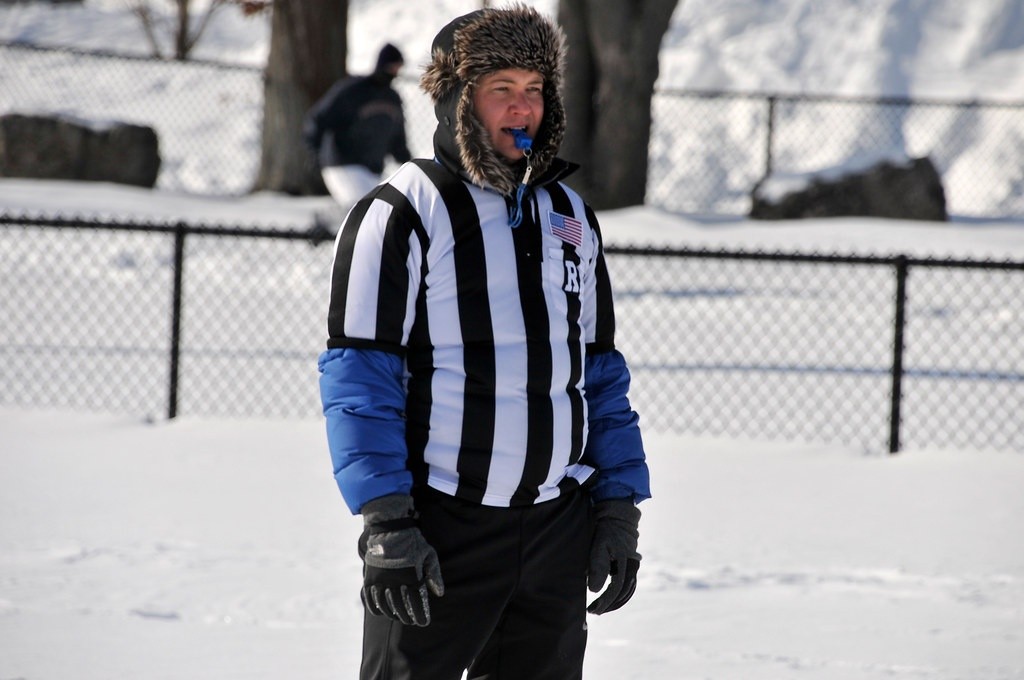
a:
[318,3,651,680]
[304,45,414,212]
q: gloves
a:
[357,494,444,628]
[588,500,643,616]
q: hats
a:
[380,45,401,63]
[422,9,567,198]
